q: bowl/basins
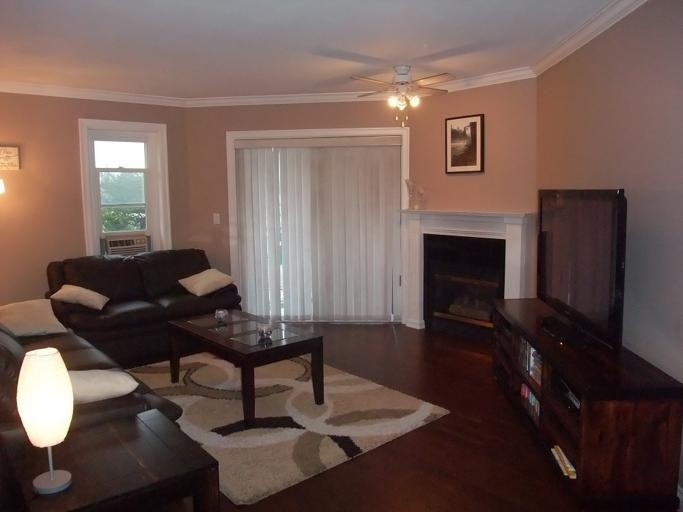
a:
[256,325,274,338]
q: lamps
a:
[388,88,420,127]
[16,348,75,495]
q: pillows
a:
[0,299,68,336]
[68,369,140,405]
[178,269,233,296]
[51,283,110,311]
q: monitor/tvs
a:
[533,187,628,357]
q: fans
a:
[351,65,456,96]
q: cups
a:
[214,308,228,323]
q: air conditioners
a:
[106,232,148,256]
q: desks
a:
[0,409,219,512]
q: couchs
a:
[0,330,182,441]
[45,248,240,365]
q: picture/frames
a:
[445,114,484,174]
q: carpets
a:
[125,353,452,505]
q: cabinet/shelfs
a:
[492,297,683,512]
